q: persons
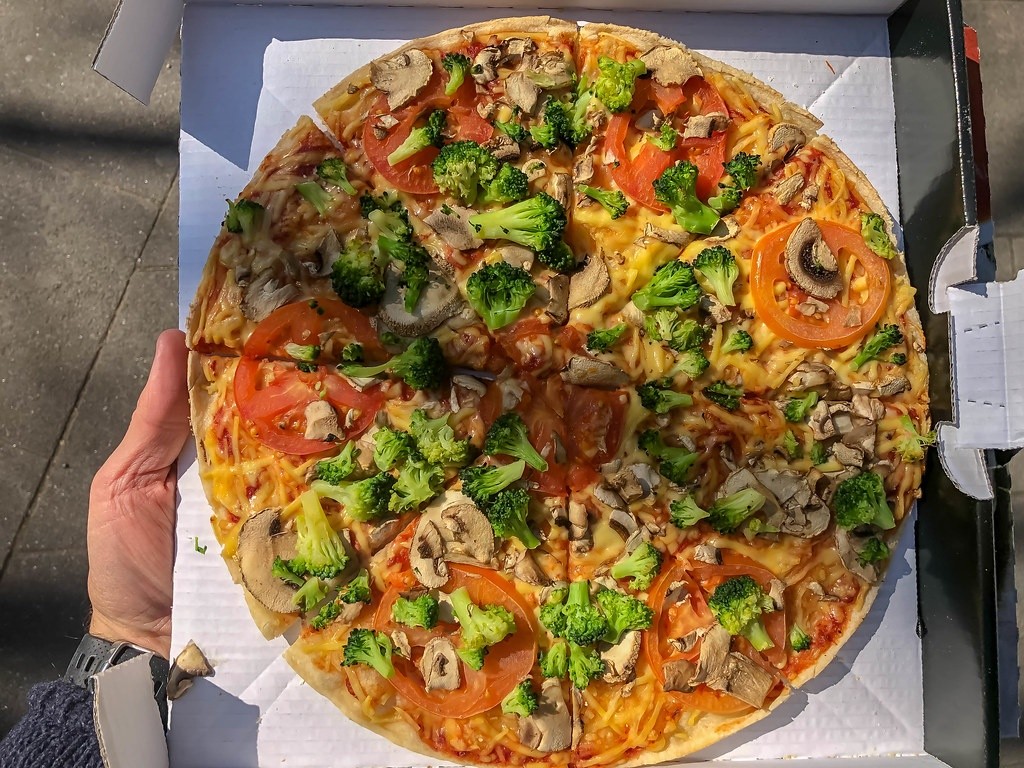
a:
[0,327,193,767]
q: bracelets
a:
[59,632,171,725]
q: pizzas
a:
[184,17,938,768]
